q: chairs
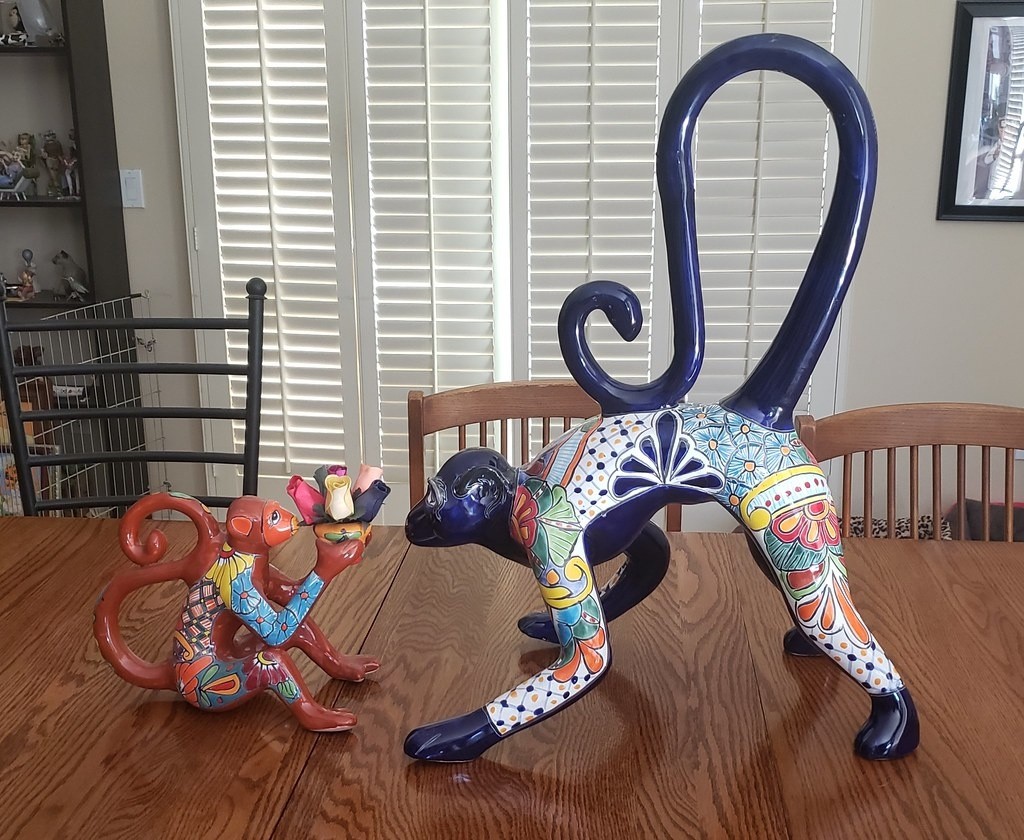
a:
[792,400,1023,544]
[1,277,268,518]
[404,381,693,532]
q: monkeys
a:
[401,30,928,765]
[92,493,381,733]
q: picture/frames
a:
[934,0,1024,222]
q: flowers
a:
[284,461,391,525]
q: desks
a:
[0,519,1024,839]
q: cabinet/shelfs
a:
[0,0,150,519]
